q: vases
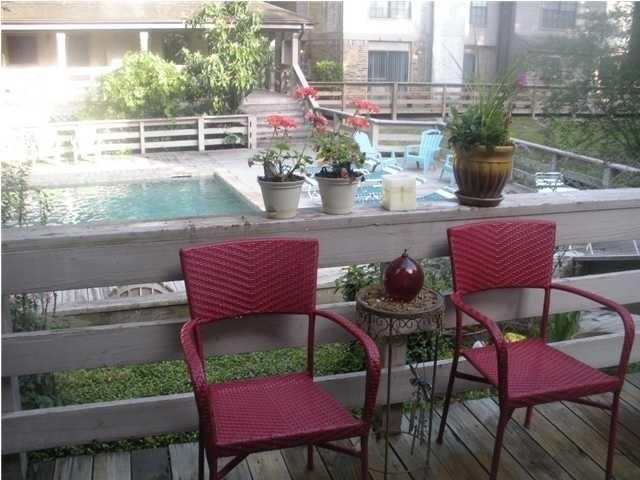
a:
[257,170,363,220]
[385,249,424,307]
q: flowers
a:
[248,86,380,182]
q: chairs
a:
[179,238,380,480]
[436,219,635,479]
[353,129,455,186]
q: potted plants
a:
[440,42,530,198]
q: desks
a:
[354,282,447,475]
